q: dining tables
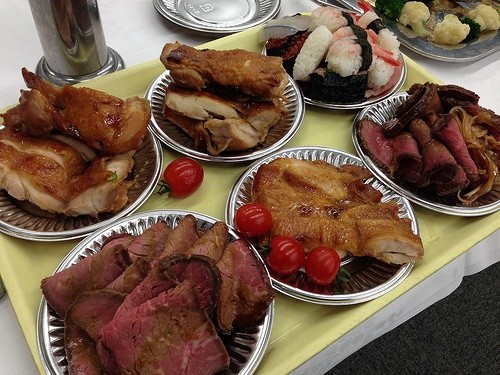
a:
[0,0,500,375]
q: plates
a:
[352,92,500,218]
[152,0,282,35]
[224,146,420,306]
[336,0,500,63]
[143,70,306,162]
[0,124,164,243]
[260,39,408,109]
[35,209,275,375]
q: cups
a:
[29,0,126,88]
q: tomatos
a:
[157,158,203,196]
[236,204,272,238]
[259,235,305,275]
[304,246,340,286]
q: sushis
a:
[261,4,400,104]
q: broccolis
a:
[375,0,500,46]
